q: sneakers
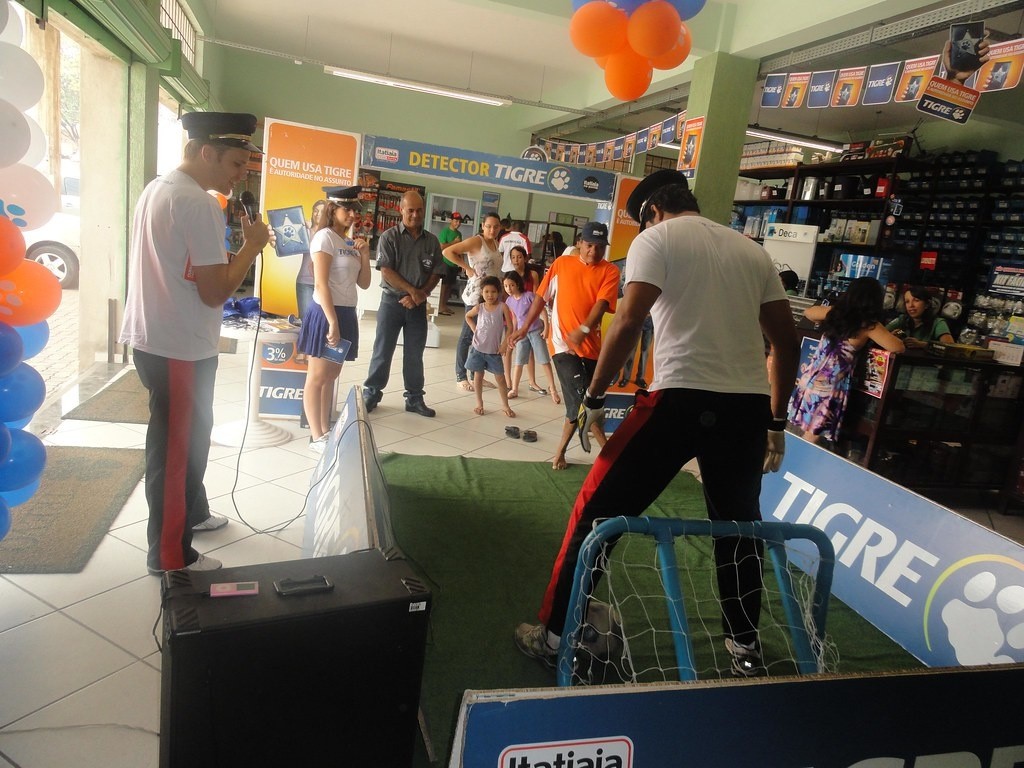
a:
[148,551,224,575]
[322,430,331,439]
[724,634,763,678]
[190,514,229,533]
[309,435,330,453]
[514,622,579,677]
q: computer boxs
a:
[158,545,432,768]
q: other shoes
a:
[439,308,455,315]
[506,387,512,394]
[481,379,497,389]
[528,385,547,394]
[458,382,474,392]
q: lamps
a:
[658,139,680,150]
[323,60,513,108]
[746,124,845,153]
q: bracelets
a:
[581,324,590,334]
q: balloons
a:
[0,1,63,544]
[571,0,707,102]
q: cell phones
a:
[273,574,335,596]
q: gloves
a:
[763,418,786,475]
[576,388,606,452]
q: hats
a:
[182,112,264,154]
[582,221,611,247]
[626,170,687,234]
[450,211,463,220]
[323,185,363,211]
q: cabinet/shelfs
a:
[424,192,480,305]
[844,342,1024,514]
[734,155,1024,303]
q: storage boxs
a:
[889,337,1024,399]
[729,135,918,286]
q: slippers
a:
[506,427,520,440]
[523,430,538,441]
[474,407,484,415]
[501,408,516,417]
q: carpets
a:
[1,443,146,574]
[60,367,151,424]
[218,336,238,353]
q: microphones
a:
[240,190,256,225]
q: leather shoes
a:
[635,377,647,388]
[406,394,435,417]
[619,378,627,387]
[362,397,377,413]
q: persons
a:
[549,231,567,262]
[442,212,503,391]
[506,221,621,470]
[502,270,561,404]
[465,276,516,418]
[884,286,955,348]
[437,212,465,315]
[296,186,370,455]
[787,276,906,446]
[495,218,511,241]
[562,232,582,255]
[267,199,326,320]
[117,113,270,576]
[505,245,547,395]
[514,168,801,676]
[778,270,798,292]
[362,191,436,418]
[498,220,531,275]
